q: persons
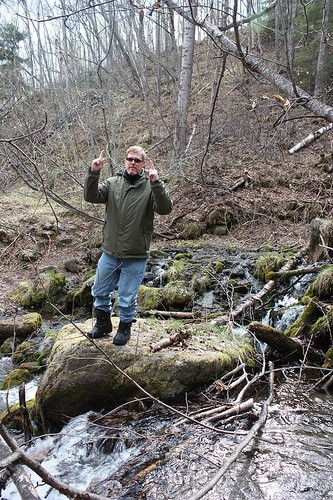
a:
[84,145,173,346]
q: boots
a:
[113,320,131,345]
[87,309,112,337]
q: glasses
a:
[126,158,142,163]
